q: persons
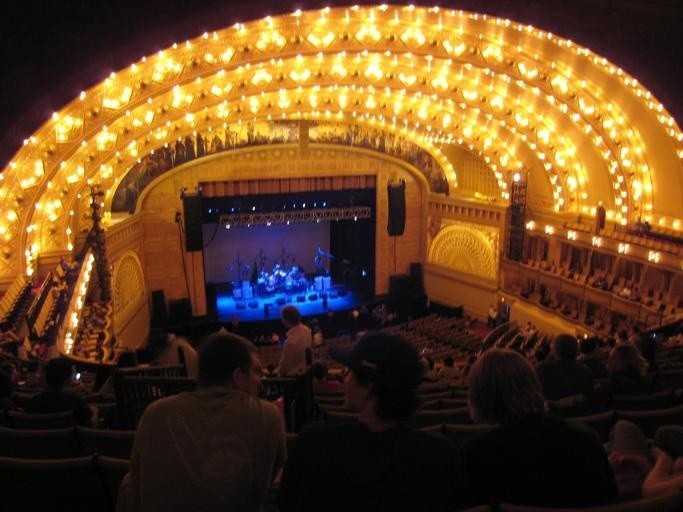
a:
[517,256,683,326]
[593,345,652,405]
[274,306,313,414]
[533,333,587,399]
[443,348,619,511]
[608,420,683,498]
[417,343,483,384]
[575,334,610,383]
[481,304,510,329]
[274,329,465,512]
[596,326,657,344]
[22,356,94,429]
[0,361,22,423]
[116,332,288,512]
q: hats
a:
[328,332,423,399]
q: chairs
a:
[615,410,683,430]
[286,432,299,453]
[565,409,614,433]
[450,381,473,389]
[446,424,498,433]
[423,399,438,409]
[2,410,73,427]
[0,425,76,460]
[553,393,587,412]
[418,389,451,399]
[453,390,471,397]
[465,503,492,512]
[78,426,139,459]
[561,237,683,333]
[407,407,473,426]
[419,424,445,433]
[65,371,115,415]
[329,412,362,419]
[613,392,682,409]
[258,345,284,373]
[362,314,483,366]
[543,399,552,414]
[0,453,98,512]
[315,390,345,397]
[416,380,448,392]
[99,455,132,512]
[315,335,353,370]
[76,301,108,361]
[319,404,353,412]
[314,396,346,405]
[441,399,467,408]
[1,347,45,407]
[485,320,551,364]
[503,490,682,512]
[508,234,563,317]
[655,342,683,385]
